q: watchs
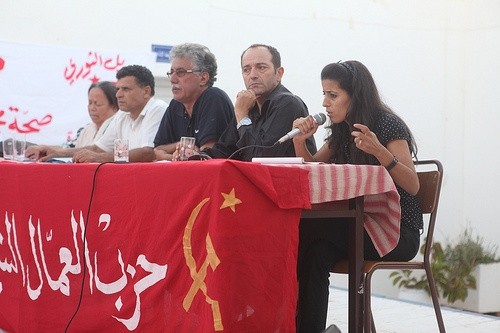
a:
[236,117,252,131]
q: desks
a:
[0,160,403,333]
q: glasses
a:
[167,69,200,78]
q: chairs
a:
[329,160,446,333]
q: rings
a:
[358,140,362,147]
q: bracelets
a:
[385,155,398,172]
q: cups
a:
[180,136,196,160]
[2,135,27,162]
[113,139,130,163]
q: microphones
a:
[278,113,326,144]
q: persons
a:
[25,81,119,159]
[292,60,423,333]
[173,44,317,162]
[153,43,237,161]
[34,65,170,163]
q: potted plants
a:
[332,222,500,314]
